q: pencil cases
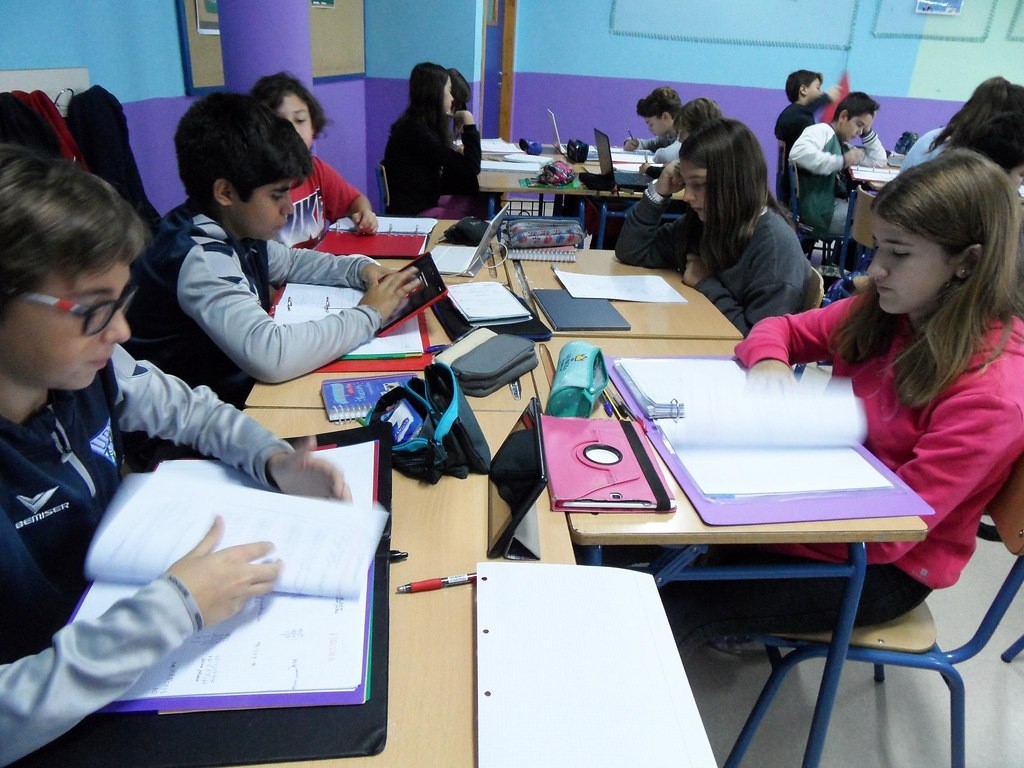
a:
[362,363,490,485]
[545,339,608,418]
[567,139,588,163]
[506,218,585,250]
[519,138,543,156]
[538,159,577,183]
[429,326,538,398]
[442,216,490,246]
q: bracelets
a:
[160,572,203,633]
[645,179,674,205]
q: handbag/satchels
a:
[368,361,492,484]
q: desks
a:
[459,143,684,250]
[502,249,745,341]
[234,406,579,768]
[531,334,928,768]
[244,284,551,413]
[312,219,510,287]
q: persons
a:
[384,62,482,220]
[658,147,1024,640]
[640,97,722,178]
[773,69,843,147]
[900,76,1024,191]
[0,143,353,768]
[120,92,421,411]
[624,87,682,164]
[790,92,887,236]
[249,70,379,250]
[617,117,812,339]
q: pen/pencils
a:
[314,441,353,450]
[395,572,477,593]
[509,378,522,401]
[426,344,449,353]
[844,142,856,149]
[614,394,647,433]
[645,149,648,164]
[389,550,408,561]
[605,387,628,418]
[627,128,636,141]
[600,391,613,417]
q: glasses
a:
[0,281,139,336]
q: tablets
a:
[487,397,547,559]
[371,252,449,337]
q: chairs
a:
[775,138,789,210]
[816,186,878,365]
[723,453,1024,768]
[787,157,853,267]
[374,158,440,218]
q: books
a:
[321,372,418,422]
[481,154,552,173]
[448,282,531,321]
[508,244,577,262]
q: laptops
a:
[547,109,598,153]
[430,202,511,276]
[594,128,655,190]
